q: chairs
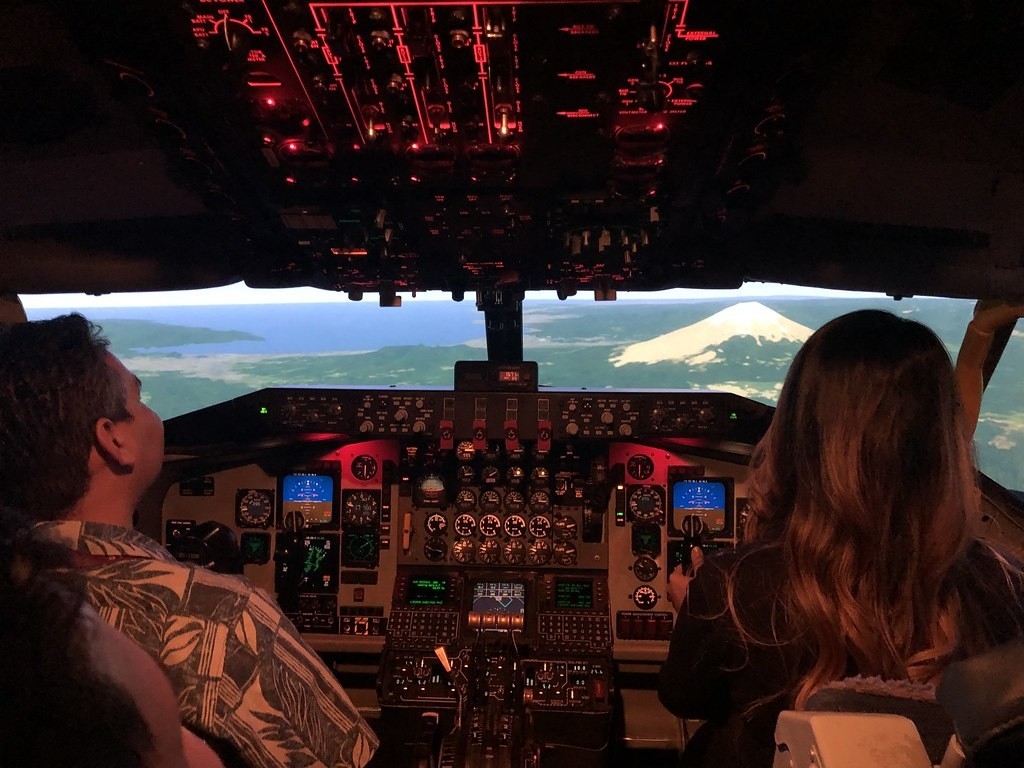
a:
[771,642,1024,768]
[0,510,227,768]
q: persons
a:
[659,309,1022,767]
[0,312,382,768]
